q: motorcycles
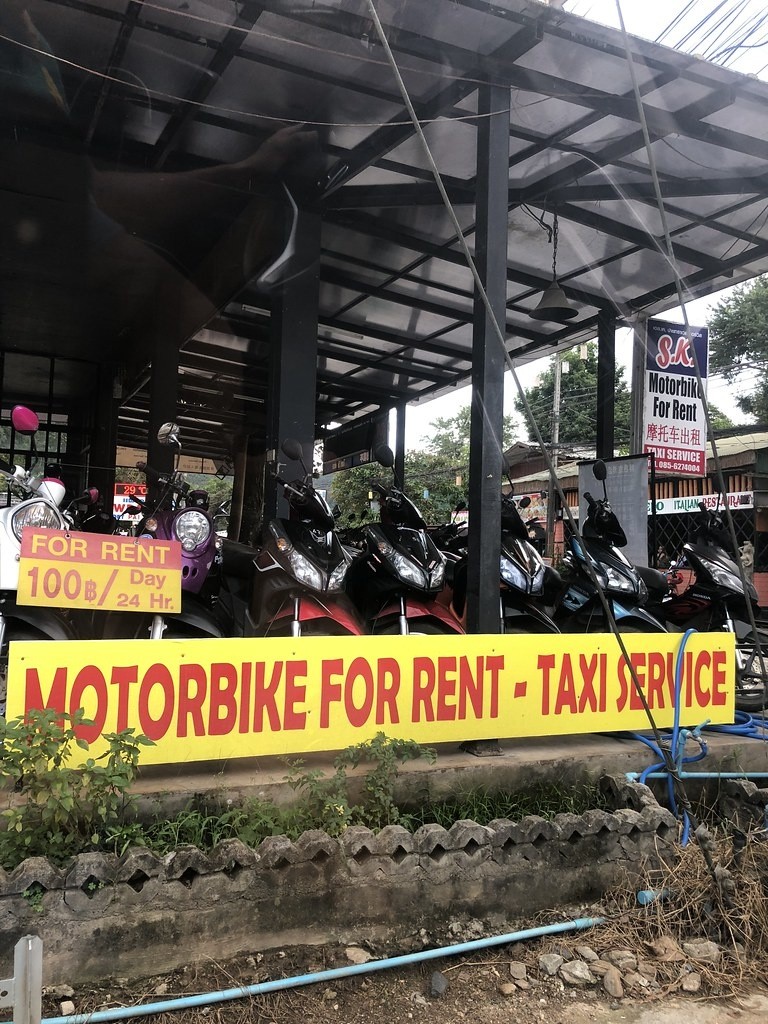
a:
[0,404,768,714]
